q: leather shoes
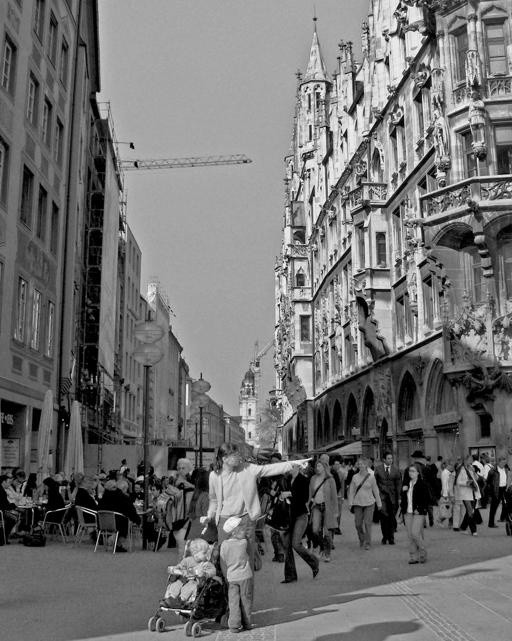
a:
[88,529,168,552]
[280,575,297,583]
[313,569,319,577]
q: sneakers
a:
[306,528,343,562]
[448,523,511,537]
[407,551,428,564]
[231,622,257,633]
[358,537,396,550]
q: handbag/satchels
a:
[264,490,290,532]
[472,506,483,524]
[350,505,354,513]
[372,502,379,523]
[439,501,452,519]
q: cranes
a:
[251,335,275,424]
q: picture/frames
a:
[0,436,20,468]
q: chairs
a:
[1,472,176,554]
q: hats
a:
[410,450,427,458]
[222,516,243,533]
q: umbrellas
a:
[62,401,84,476]
[36,390,54,485]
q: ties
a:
[385,467,389,479]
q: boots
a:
[272,551,284,563]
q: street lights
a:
[191,414,200,468]
[132,310,164,523]
[191,374,212,471]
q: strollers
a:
[145,520,229,638]
